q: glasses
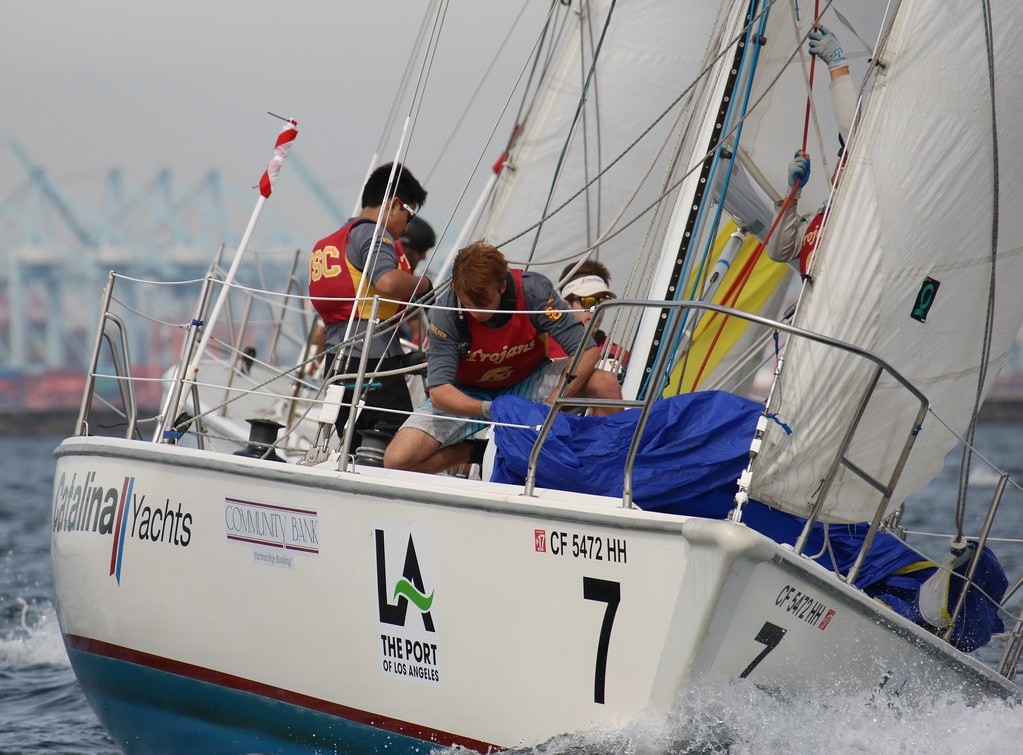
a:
[567,296,612,308]
[398,198,418,223]
[838,148,846,156]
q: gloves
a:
[415,275,436,311]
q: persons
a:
[766,23,858,287]
[380,234,623,480]
[544,258,632,368]
[308,161,437,464]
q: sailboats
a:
[52,0,1022,754]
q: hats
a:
[839,133,848,146]
[561,275,618,300]
